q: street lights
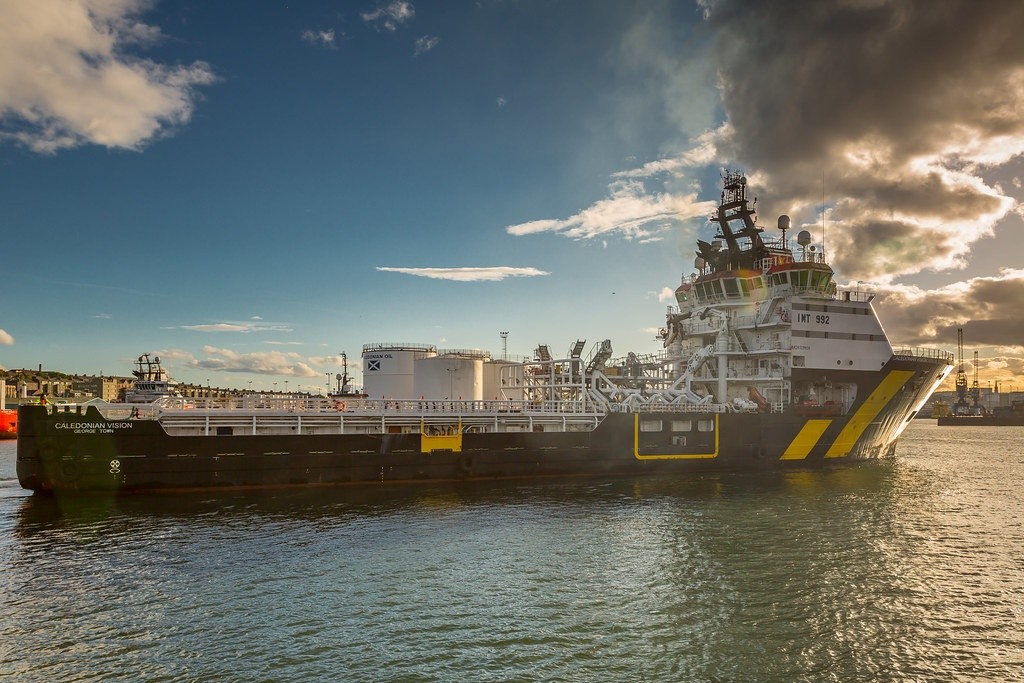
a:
[999,383,1001,402]
[273,383,277,393]
[988,381,990,409]
[284,381,288,396]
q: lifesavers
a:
[335,401,345,412]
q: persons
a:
[34,393,55,405]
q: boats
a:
[124,353,187,404]
[17,166,959,493]
